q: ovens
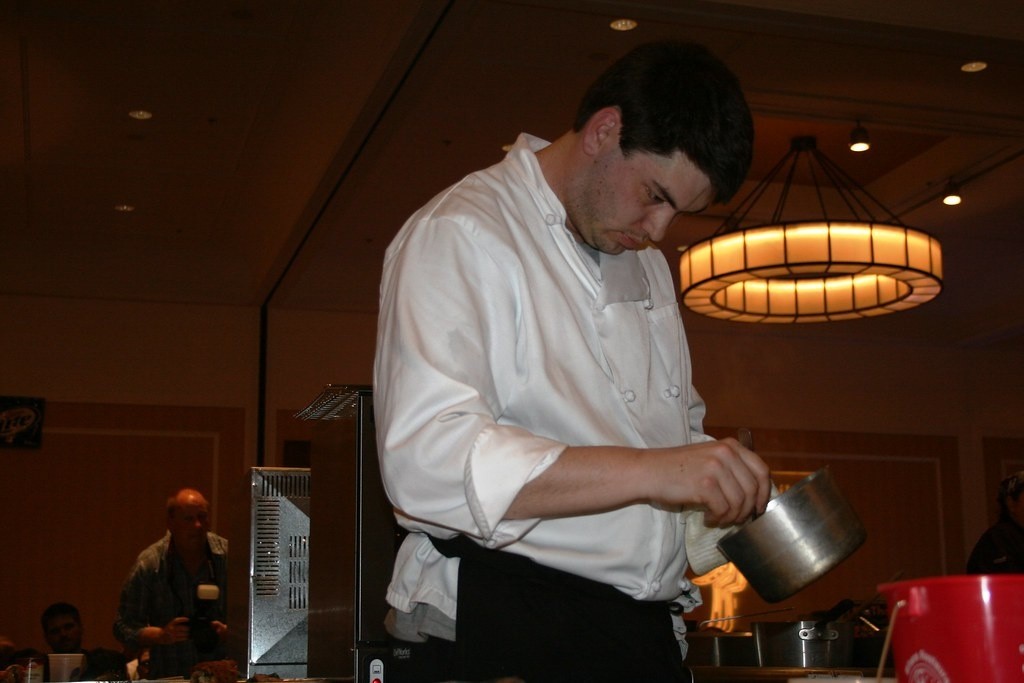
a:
[243,394,450,682]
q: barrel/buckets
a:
[685,632,754,664]
[750,600,882,669]
[877,574,1024,682]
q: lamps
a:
[943,175,962,205]
[678,136,943,324]
[848,123,871,152]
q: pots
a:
[716,468,867,603]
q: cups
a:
[5,658,44,683]
[48,654,85,682]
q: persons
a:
[371,38,773,683]
[136,647,150,679]
[41,602,126,682]
[967,471,1024,574]
[113,489,230,679]
